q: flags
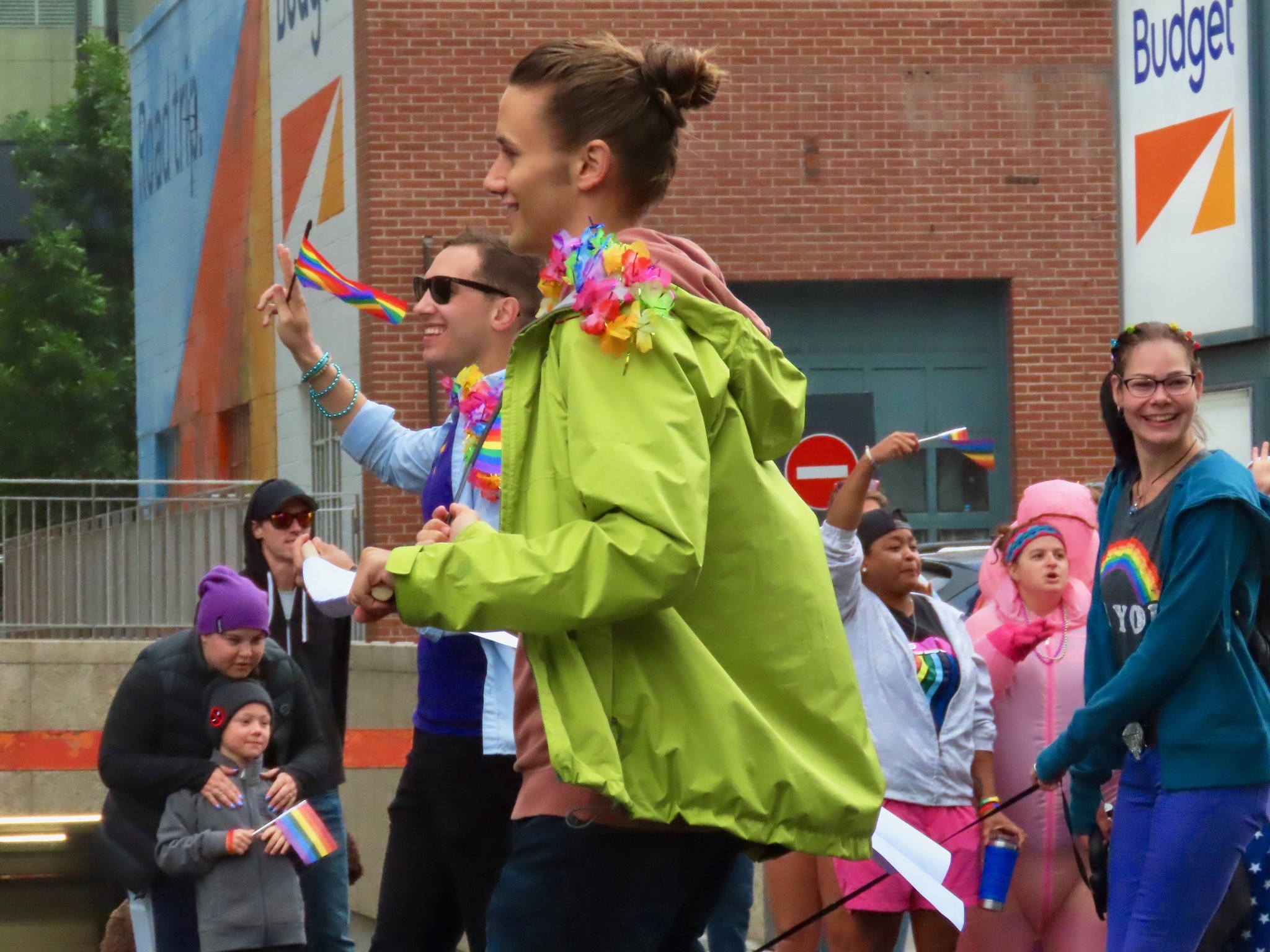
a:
[939,429,995,471]
[293,236,408,325]
[276,803,340,866]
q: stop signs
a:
[784,433,859,512]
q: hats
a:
[193,566,271,640]
[858,507,913,547]
[253,479,320,520]
[206,678,275,751]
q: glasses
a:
[1114,372,1197,398]
[263,508,315,530]
[831,478,881,492]
[411,274,522,319]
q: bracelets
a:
[300,352,358,418]
[864,444,881,470]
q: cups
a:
[977,833,1018,913]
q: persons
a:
[257,232,549,952]
[97,565,343,952]
[154,680,306,952]
[350,40,880,952]
[238,478,358,952]
[709,321,1270,952]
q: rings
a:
[289,792,294,798]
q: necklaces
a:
[908,594,916,643]
[1128,439,1197,516]
[1023,599,1069,660]
[1019,599,1066,665]
